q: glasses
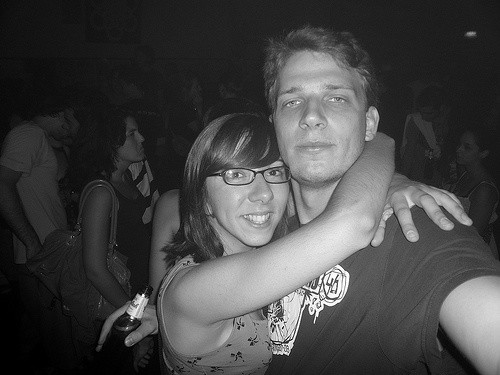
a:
[206,167,292,186]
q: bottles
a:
[94,284,154,375]
[424,149,434,178]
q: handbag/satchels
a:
[25,180,132,320]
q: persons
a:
[155,115,474,375]
[0,46,500,375]
[95,25,500,375]
